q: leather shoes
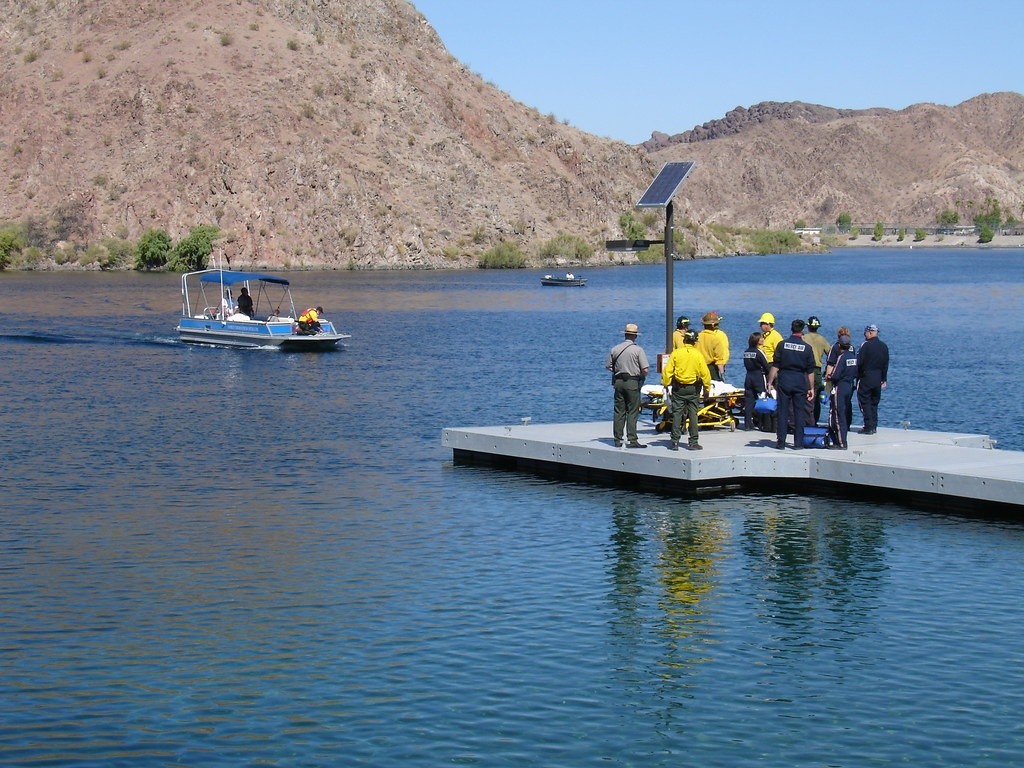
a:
[625,440,647,448]
[614,440,623,447]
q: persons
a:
[826,325,853,431]
[802,317,831,424]
[767,320,814,450]
[743,332,768,430]
[662,330,711,450]
[605,323,648,448]
[223,289,238,314]
[672,317,690,350]
[295,306,324,335]
[708,311,729,367]
[828,334,858,451]
[758,312,782,369]
[855,325,890,434]
[237,287,255,319]
[566,272,574,280]
[694,314,724,382]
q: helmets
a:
[682,329,699,341]
[757,313,774,324]
[701,313,722,325]
[675,316,692,325]
[806,317,821,327]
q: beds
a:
[640,385,747,431]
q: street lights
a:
[606,240,673,355]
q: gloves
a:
[719,373,725,382]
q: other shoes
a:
[776,444,785,449]
[667,443,679,449]
[794,445,804,449]
[874,428,876,432]
[858,428,872,434]
[689,443,702,449]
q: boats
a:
[174,248,352,351]
[540,278,587,287]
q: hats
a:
[839,335,851,346]
[318,306,323,313]
[620,324,641,335]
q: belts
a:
[614,376,640,380]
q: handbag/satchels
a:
[820,391,830,404]
[757,375,778,399]
[803,427,833,448]
[754,393,777,413]
